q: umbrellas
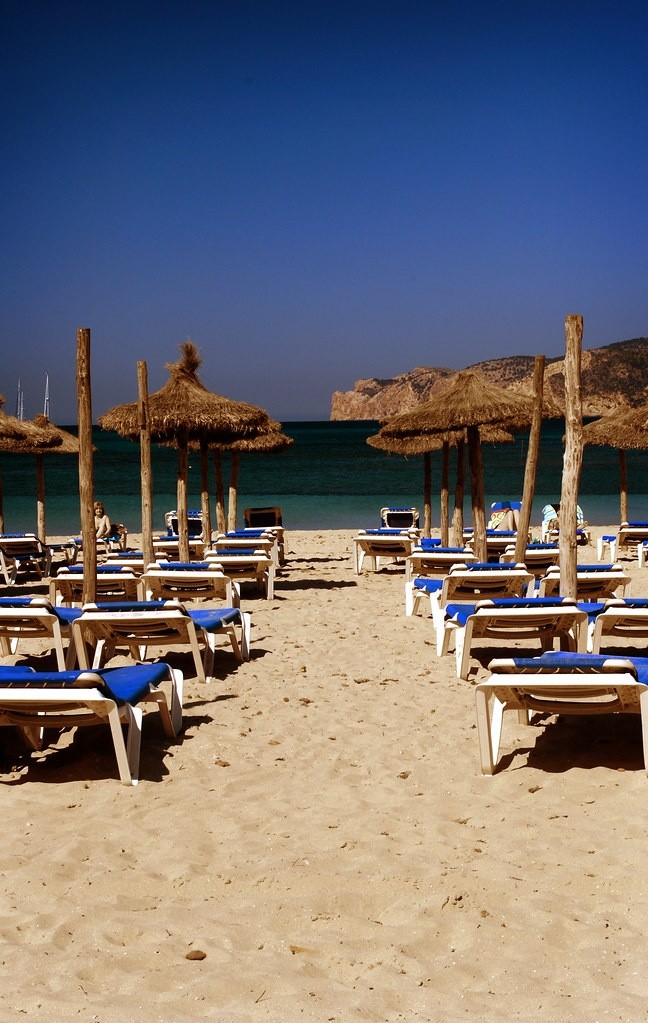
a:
[95,342,293,565]
[0,396,98,543]
[368,374,553,563]
[563,398,647,448]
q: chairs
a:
[352,501,648,776]
[0,508,286,785]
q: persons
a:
[492,507,519,531]
[548,509,588,530]
[93,502,112,538]
